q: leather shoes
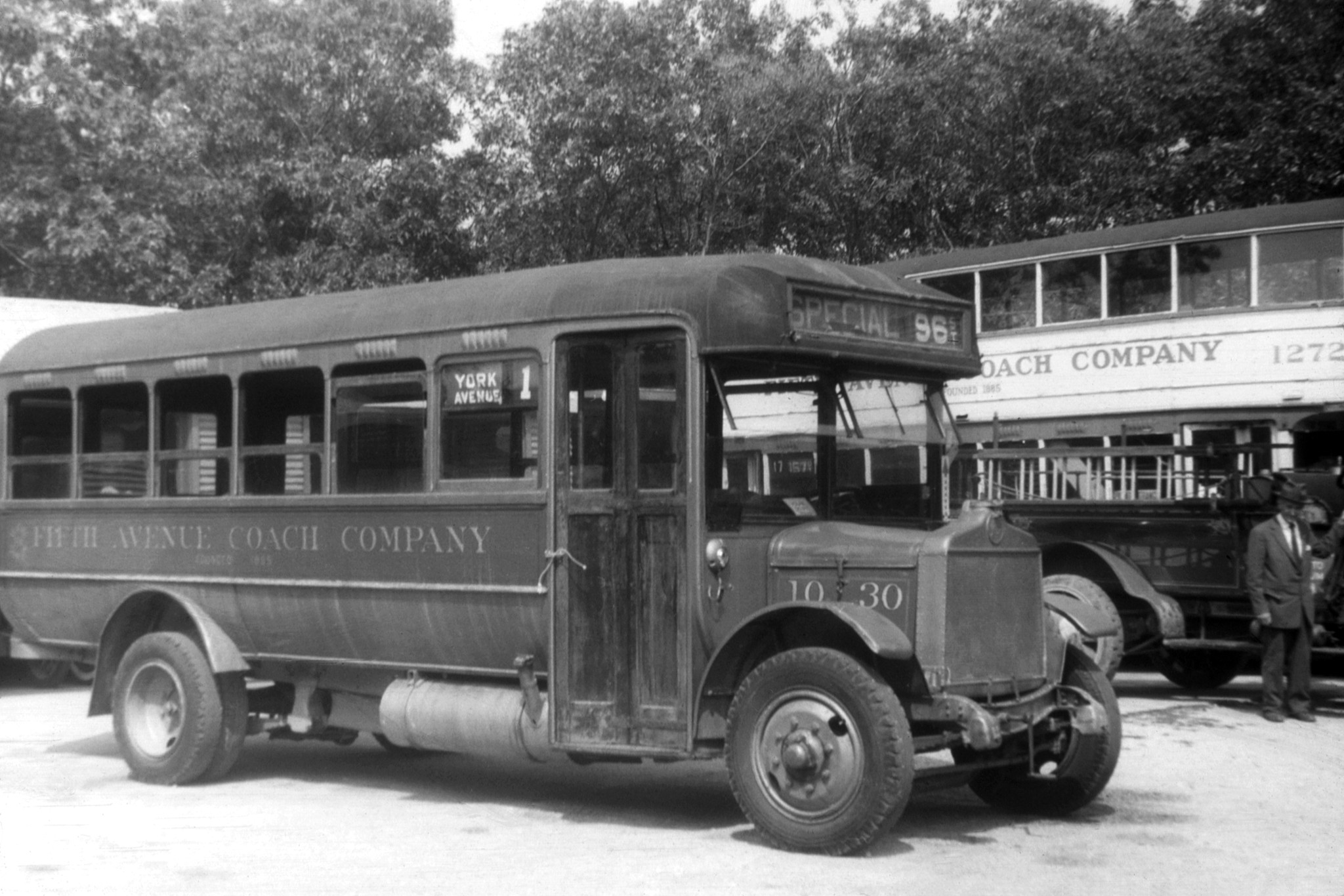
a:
[1290,708,1316,722]
[1264,708,1284,723]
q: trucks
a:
[956,434,1344,706]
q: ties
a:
[1287,524,1302,561]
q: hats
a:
[1274,478,1316,504]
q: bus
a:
[721,198,1334,501]
[3,253,1125,859]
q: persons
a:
[1247,478,1344,723]
